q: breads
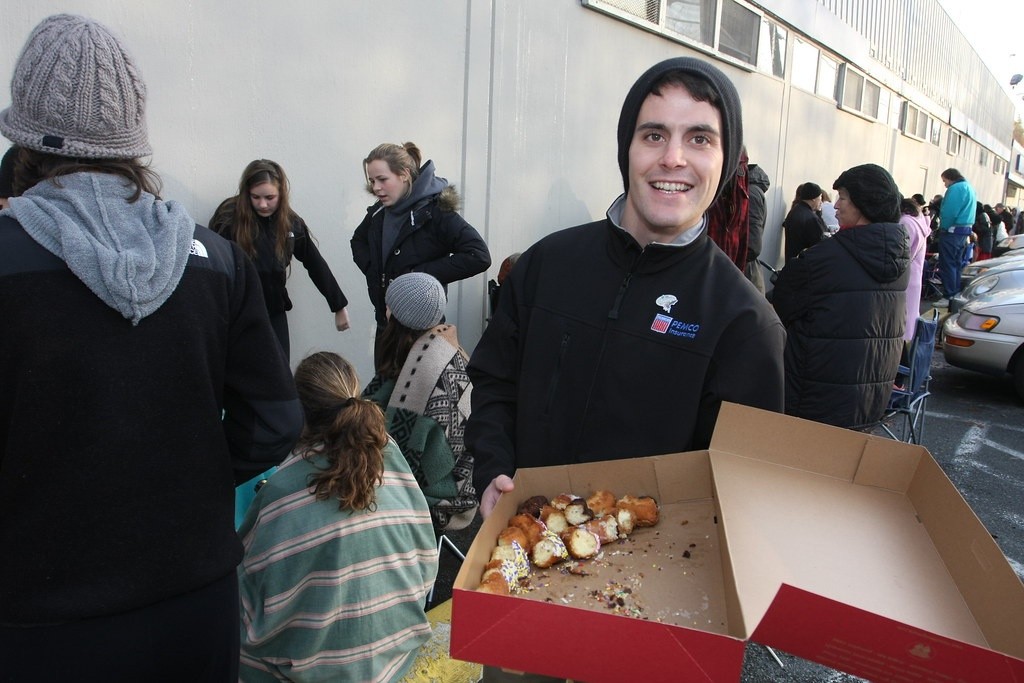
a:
[476,491,661,596]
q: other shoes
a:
[931,298,949,308]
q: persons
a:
[238,351,440,683]
[463,58,786,520]
[765,163,911,430]
[0,13,304,682]
[208,159,348,369]
[910,168,976,307]
[782,182,838,264]
[893,194,931,392]
[708,145,770,297]
[357,272,477,530]
[971,201,1024,260]
[351,143,492,372]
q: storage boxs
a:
[448,401,1023,682]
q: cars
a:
[940,233,1024,387]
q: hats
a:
[617,57,743,209]
[0,14,153,157]
[832,164,901,224]
[385,272,446,331]
[801,182,822,200]
[913,194,926,205]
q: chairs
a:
[863,307,939,445]
[924,249,945,299]
